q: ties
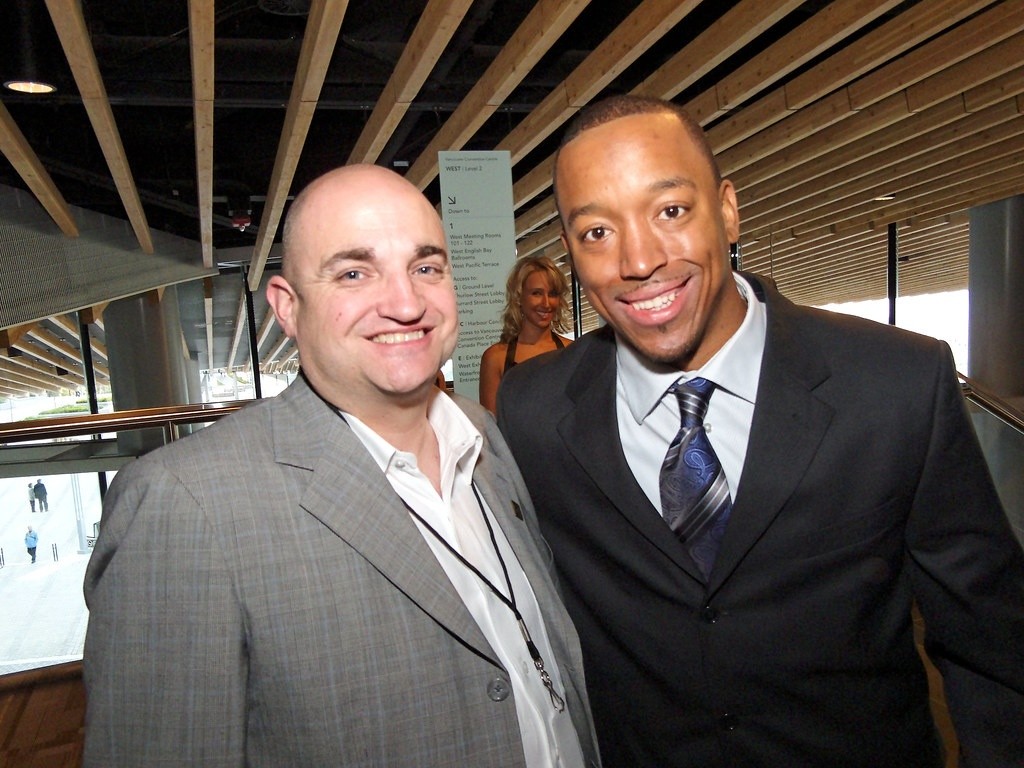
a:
[659,377,733,585]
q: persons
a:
[28,480,48,513]
[82,164,603,768]
[480,92,1024,768]
[24,526,38,564]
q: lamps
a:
[3,0,59,94]
